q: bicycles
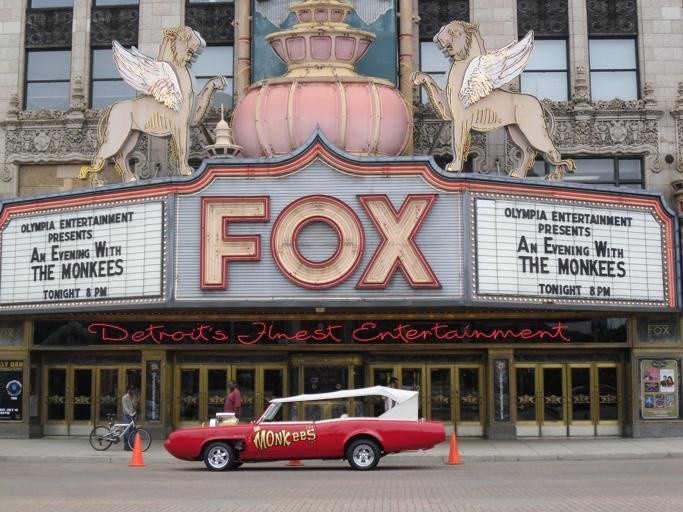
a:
[86,410,152,452]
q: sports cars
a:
[164,382,446,471]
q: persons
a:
[121,385,140,451]
[643,368,674,393]
[222,380,241,418]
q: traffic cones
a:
[442,430,463,466]
[127,429,148,467]
[285,458,303,469]
